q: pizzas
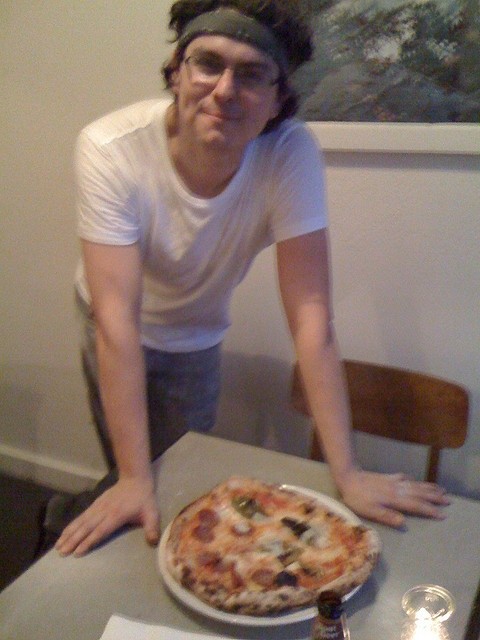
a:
[166,474,379,615]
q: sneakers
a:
[34,494,72,560]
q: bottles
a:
[312,589,349,640]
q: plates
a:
[158,483,362,627]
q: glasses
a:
[177,48,281,92]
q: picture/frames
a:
[288,0,480,159]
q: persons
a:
[30,0,453,562]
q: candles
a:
[409,601,443,640]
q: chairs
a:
[292,353,470,490]
